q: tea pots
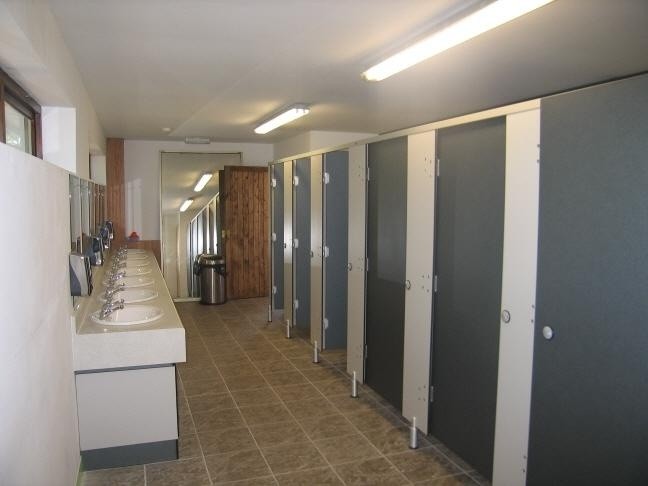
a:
[160,149,242,306]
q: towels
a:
[198,254,226,305]
[193,255,200,297]
